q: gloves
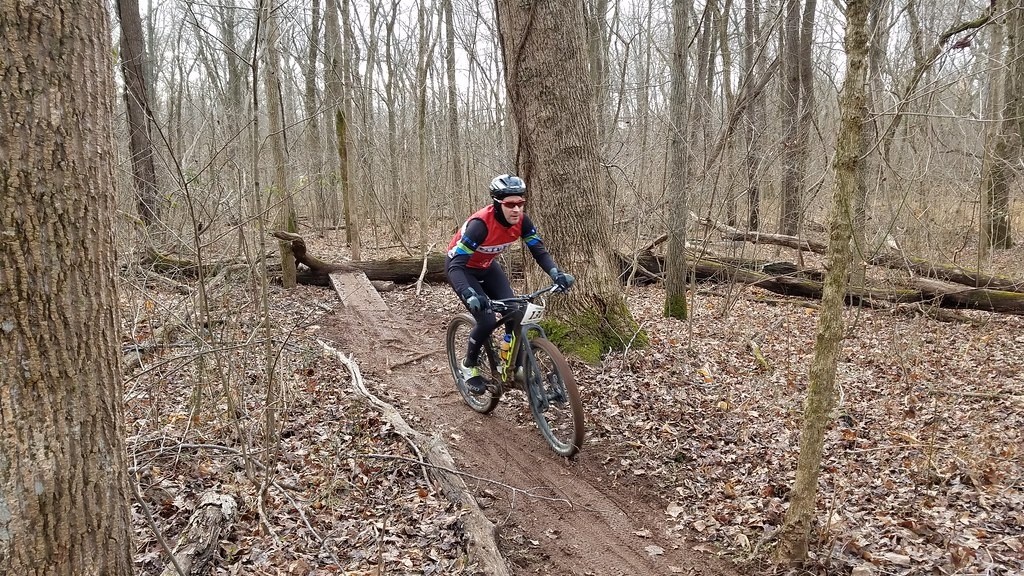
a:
[549,268,575,294]
[462,287,492,314]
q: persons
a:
[443,175,577,395]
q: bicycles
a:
[445,282,586,458]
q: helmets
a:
[488,174,526,197]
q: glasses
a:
[493,198,527,208]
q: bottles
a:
[501,334,512,360]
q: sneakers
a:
[460,358,486,394]
